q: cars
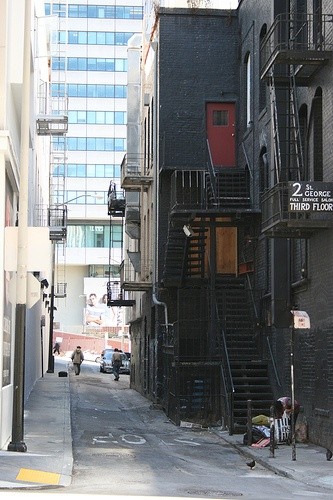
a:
[99,348,131,375]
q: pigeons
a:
[245,460,256,469]
[326,447,332,461]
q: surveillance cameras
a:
[183,225,194,237]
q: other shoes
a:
[114,377,120,381]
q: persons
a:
[112,348,122,381]
[85,292,125,327]
[53,343,60,355]
[71,345,85,375]
[270,397,301,446]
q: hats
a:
[77,346,81,348]
